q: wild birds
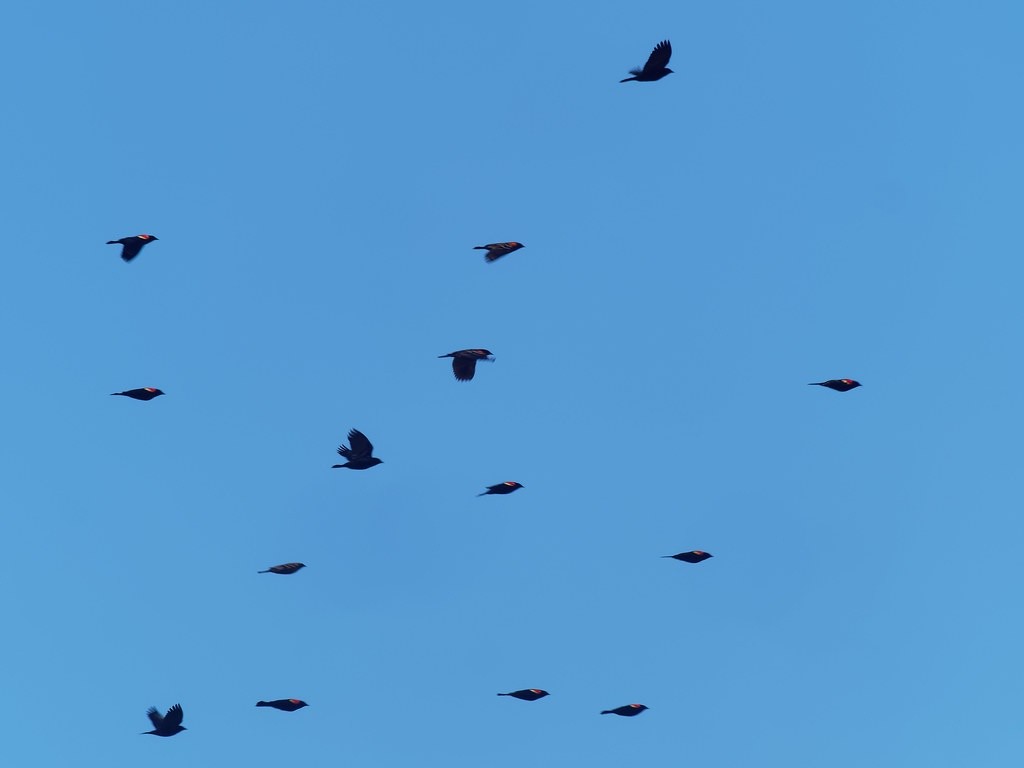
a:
[330,426,385,471]
[110,386,166,401]
[104,233,160,264]
[476,481,525,497]
[472,241,526,264]
[138,702,189,738]
[254,698,310,713]
[496,688,553,703]
[437,347,497,383]
[659,549,716,565]
[807,379,863,393]
[256,561,308,576]
[617,37,676,84]
[599,702,652,718]
